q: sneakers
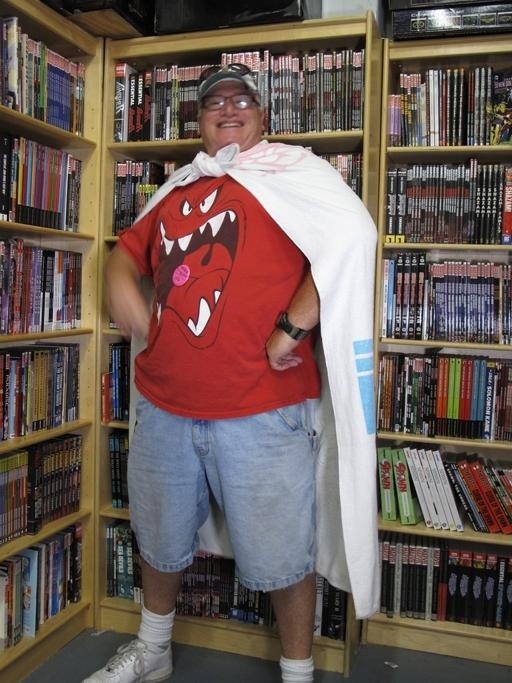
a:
[82,638,173,682]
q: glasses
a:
[201,93,254,110]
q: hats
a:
[196,62,258,97]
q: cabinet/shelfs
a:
[0,0,104,682]
[374,29,511,670]
